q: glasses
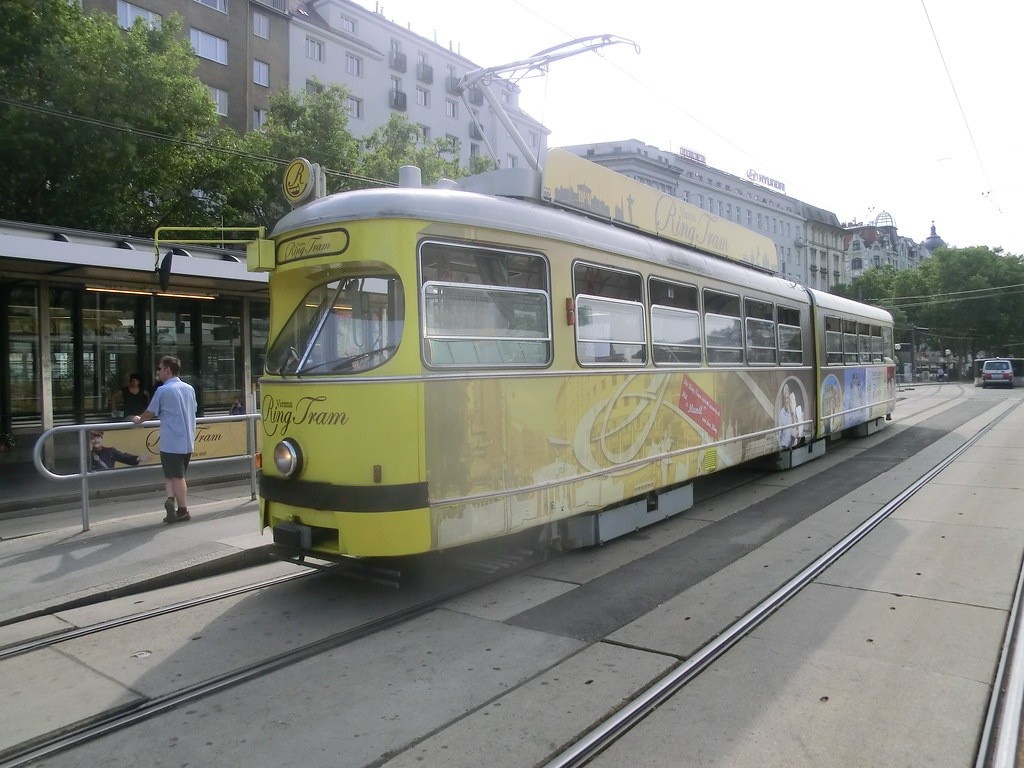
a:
[158,367,166,371]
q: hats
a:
[90,431,104,438]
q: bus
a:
[257,32,896,594]
[5,306,245,417]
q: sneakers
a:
[163,499,190,523]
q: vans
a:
[982,360,1014,388]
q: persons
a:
[150,367,165,403]
[788,390,805,446]
[132,355,198,523]
[89,431,155,471]
[942,371,948,382]
[109,372,151,423]
[829,392,842,433]
[937,366,944,382]
[779,384,796,449]
[894,357,903,383]
[229,396,243,421]
